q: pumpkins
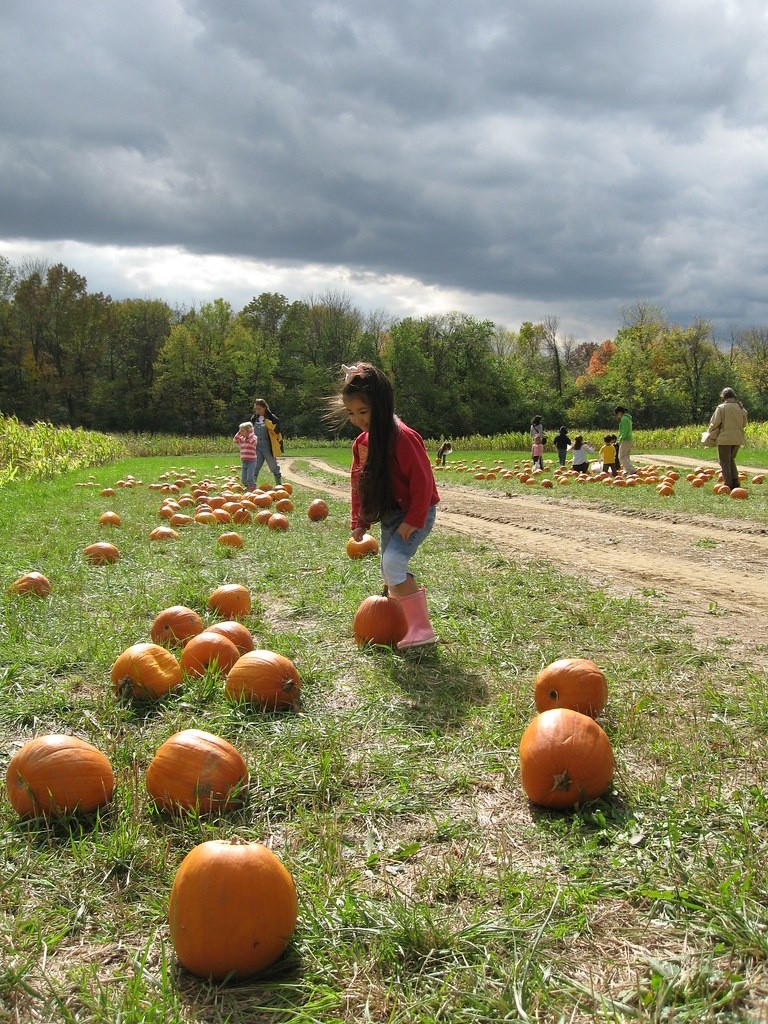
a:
[533,658,608,721]
[9,571,51,597]
[519,707,615,808]
[6,735,114,817]
[168,833,297,981]
[110,585,302,712]
[73,465,378,565]
[429,456,765,500]
[144,729,247,816]
[353,584,408,648]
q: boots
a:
[254,475,257,485]
[397,584,436,649]
[274,474,281,486]
[248,486,255,493]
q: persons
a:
[531,415,546,470]
[599,434,621,477]
[554,426,572,466]
[251,399,285,485]
[438,443,451,467]
[615,406,639,476]
[708,387,747,491]
[320,363,441,648]
[572,435,595,474]
[233,422,258,493]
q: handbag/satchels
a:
[541,438,547,445]
